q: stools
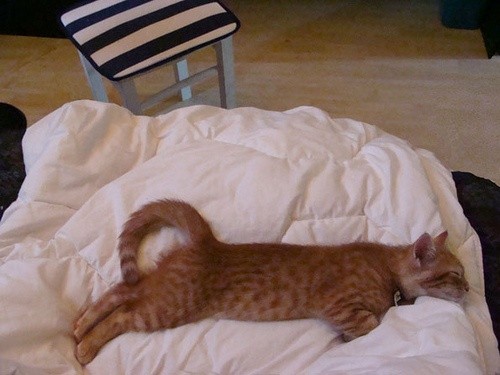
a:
[61,0,241,117]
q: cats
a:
[72,195,470,366]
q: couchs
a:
[0,100,500,375]
